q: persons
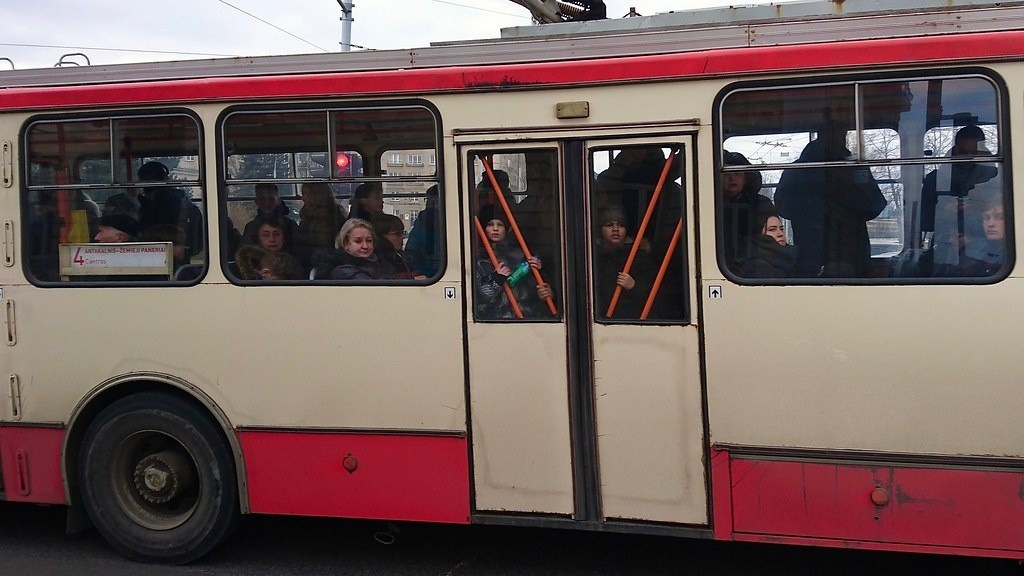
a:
[226,182,441,280]
[592,146,682,320]
[25,161,202,280]
[923,126,1005,277]
[472,170,561,318]
[716,119,888,279]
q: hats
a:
[107,193,141,223]
[478,205,509,231]
[90,215,138,237]
[597,203,628,227]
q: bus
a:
[1,1,1023,576]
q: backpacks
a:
[166,189,203,256]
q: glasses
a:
[945,233,965,239]
[182,244,190,250]
[423,198,436,204]
[384,231,406,237]
